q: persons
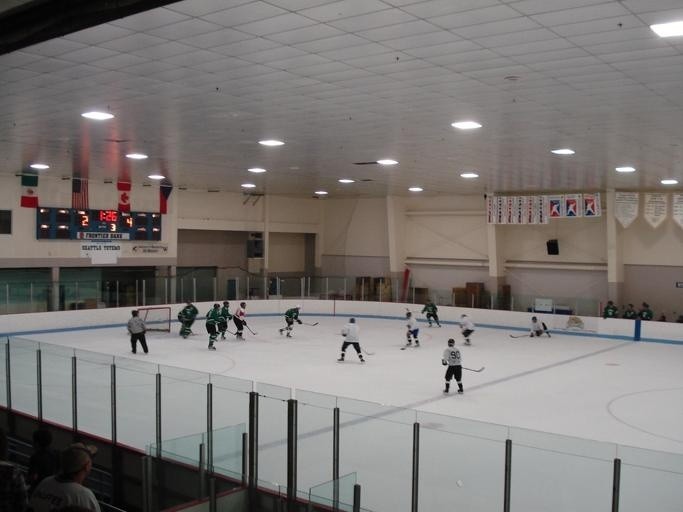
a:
[405,307,420,346]
[604,301,652,321]
[178,301,247,350]
[459,313,475,344]
[337,318,365,362]
[531,316,552,337]
[279,306,303,337]
[0,427,99,511]
[442,339,463,393]
[128,310,149,353]
[422,297,442,327]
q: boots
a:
[428,320,432,326]
[357,353,367,362]
[458,383,464,392]
[436,320,441,326]
[407,339,419,345]
[337,352,346,362]
[279,328,285,335]
[286,331,293,337]
[178,329,245,349]
[443,382,449,393]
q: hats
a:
[63,442,97,472]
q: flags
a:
[160,185,173,214]
[20,174,38,209]
[71,178,89,209]
[117,181,131,213]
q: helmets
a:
[448,338,455,345]
[295,304,301,309]
[406,312,411,317]
[350,317,355,322]
[186,299,246,309]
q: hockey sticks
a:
[510,329,552,338]
[302,322,319,326]
[461,366,485,372]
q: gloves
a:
[442,359,448,366]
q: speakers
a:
[547,239,559,255]
[247,240,263,258]
